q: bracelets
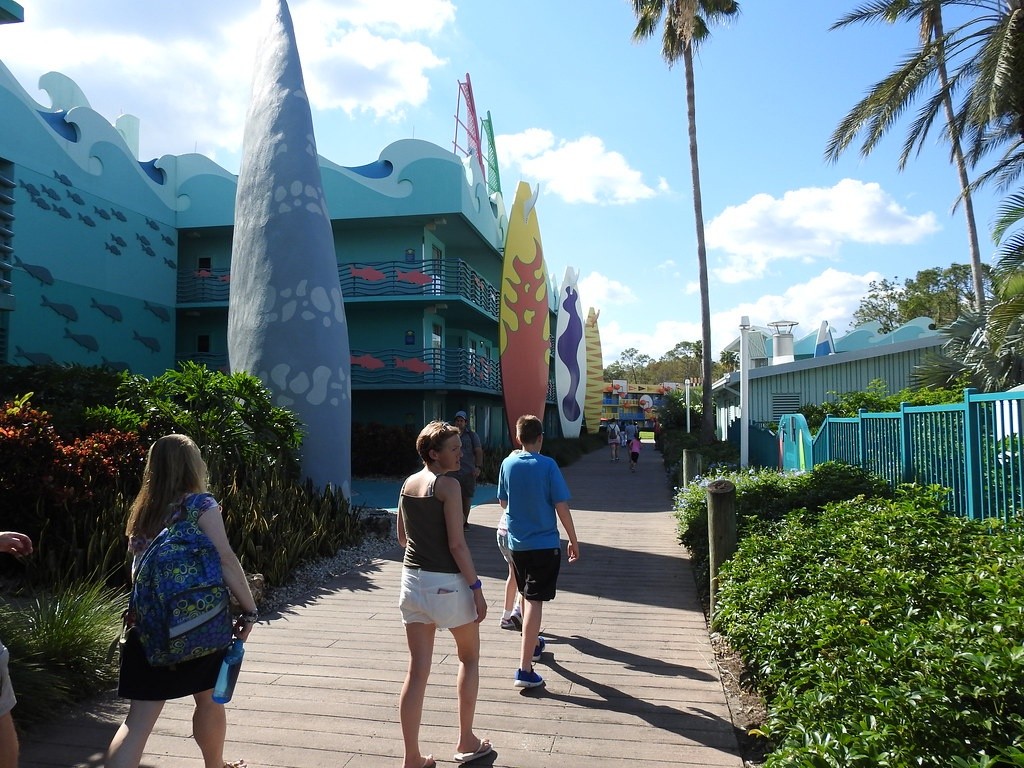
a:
[475,465,482,472]
[470,579,482,590]
[239,609,258,623]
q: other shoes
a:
[500,617,516,628]
[615,457,620,461]
[463,521,470,531]
[628,460,632,463]
[510,609,523,631]
[611,459,615,463]
[633,464,636,468]
[631,467,635,472]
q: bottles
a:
[210,638,245,705]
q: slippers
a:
[454,738,492,761]
[421,754,436,768]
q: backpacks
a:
[608,424,618,439]
[120,492,233,667]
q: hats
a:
[455,411,467,420]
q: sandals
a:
[224,760,246,768]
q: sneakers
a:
[514,664,543,687]
[532,635,545,661]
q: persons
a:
[497,450,523,629]
[497,416,579,688]
[625,421,639,465]
[607,417,620,463]
[619,421,627,447]
[0,531,33,768]
[454,411,483,532]
[629,433,641,473]
[397,421,493,768]
[104,434,258,768]
